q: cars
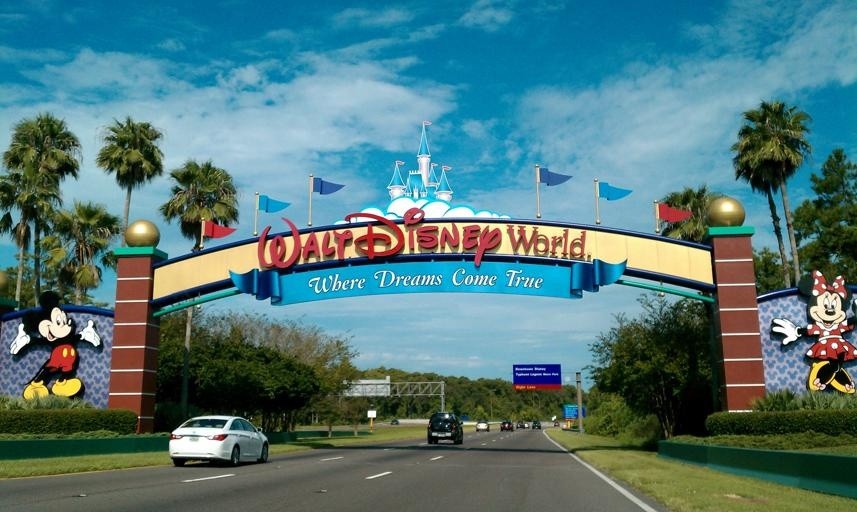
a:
[390,420,399,426]
[476,420,560,431]
[167,412,271,467]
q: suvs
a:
[427,412,464,445]
